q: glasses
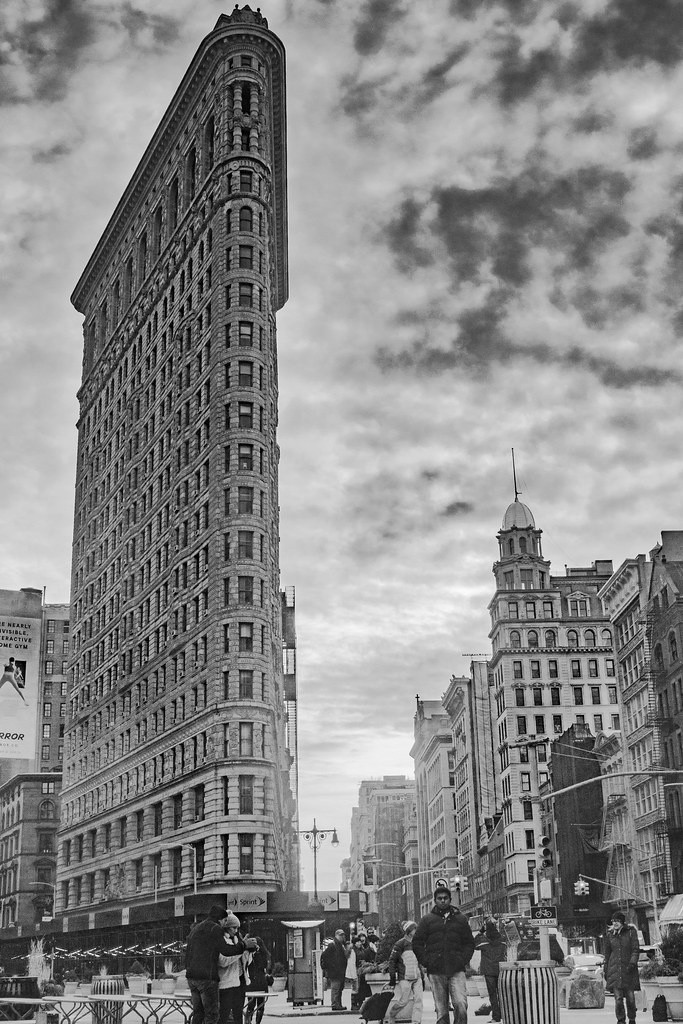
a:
[369,932,374,934]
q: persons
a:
[474,918,504,1023]
[604,913,641,1024]
[246,937,272,1024]
[0,657,29,706]
[184,905,259,1024]
[383,921,423,1024]
[411,887,476,1024]
[323,929,379,1010]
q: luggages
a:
[360,982,395,1024]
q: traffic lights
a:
[460,876,468,892]
[574,881,580,895]
[584,882,590,895]
[536,835,551,870]
[451,875,460,892]
[580,882,586,896]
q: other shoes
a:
[488,1019,501,1024]
[332,1006,346,1011]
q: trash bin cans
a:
[0,977,41,1021]
[498,960,560,1024]
[91,975,125,1024]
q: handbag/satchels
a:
[265,974,274,986]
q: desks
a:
[0,991,279,1024]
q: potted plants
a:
[639,958,683,1022]
[465,966,488,998]
[158,973,180,993]
[358,960,398,995]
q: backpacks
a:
[652,994,668,1021]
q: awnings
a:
[658,894,683,924]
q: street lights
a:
[160,843,197,922]
[293,828,340,901]
[29,881,56,980]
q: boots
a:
[351,993,360,1010]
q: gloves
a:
[388,975,396,985]
[628,964,635,972]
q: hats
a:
[351,937,361,944]
[223,909,240,927]
[335,929,345,936]
[369,935,379,943]
[401,921,417,933]
[612,912,625,925]
[208,906,226,920]
